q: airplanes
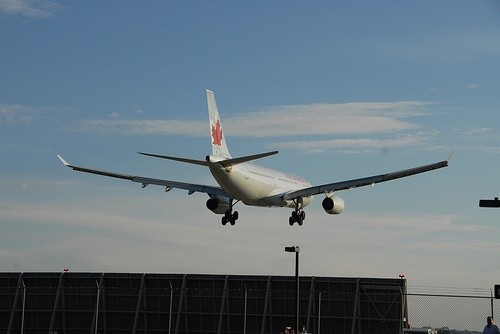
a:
[57,89,449,226]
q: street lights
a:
[285,246,300,334]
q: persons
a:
[482,316,500,334]
